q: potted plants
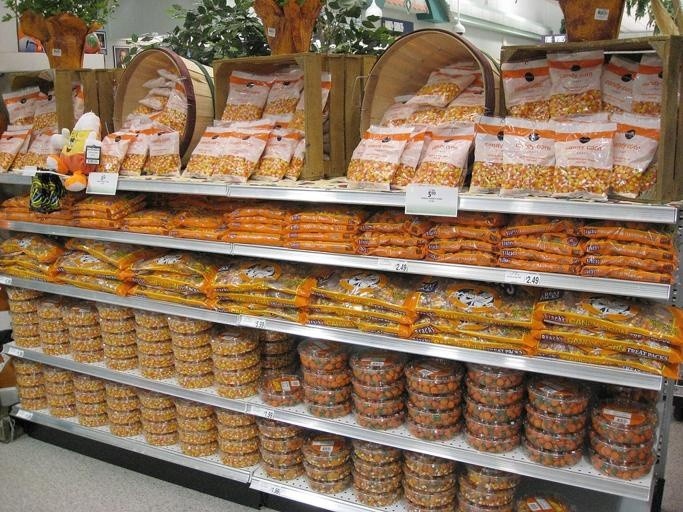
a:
[557,0,649,42]
[0,0,119,70]
[251,1,326,56]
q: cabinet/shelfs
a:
[0,172,683,512]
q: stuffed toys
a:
[47,112,102,192]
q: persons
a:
[117,51,127,69]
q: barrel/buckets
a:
[351,29,505,187]
[111,46,215,169]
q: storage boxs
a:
[0,69,125,140]
[213,55,377,180]
